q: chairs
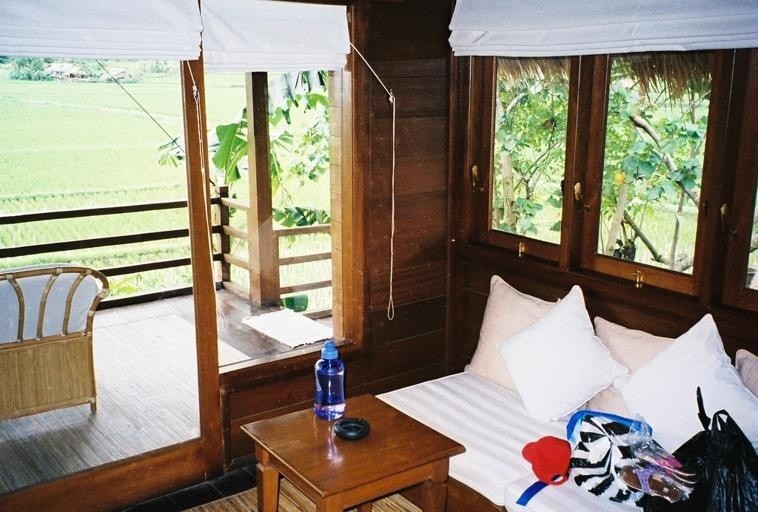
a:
[0,263,109,420]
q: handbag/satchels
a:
[569,415,640,504]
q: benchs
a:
[371,237,757,511]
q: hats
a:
[523,436,571,486]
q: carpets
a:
[240,307,333,350]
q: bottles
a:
[314,340,346,421]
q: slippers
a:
[629,441,699,487]
[620,464,680,502]
